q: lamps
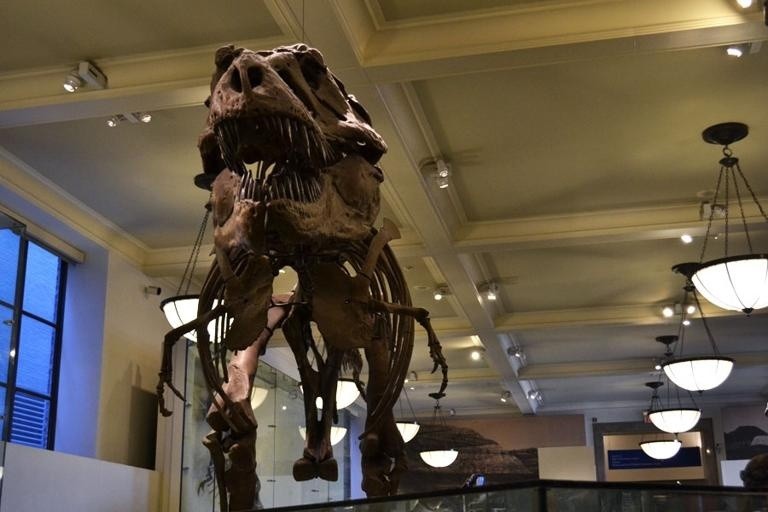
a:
[159,187,236,348]
[62,60,107,93]
[421,156,451,189]
[107,110,153,129]
